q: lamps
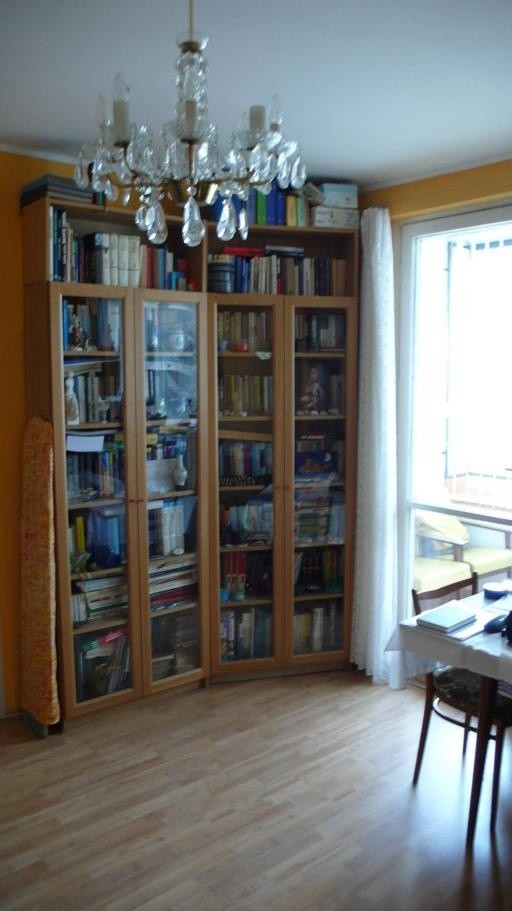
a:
[69,0,311,250]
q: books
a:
[416,615,495,641]
[416,600,478,633]
[19,163,361,703]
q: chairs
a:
[410,566,512,834]
